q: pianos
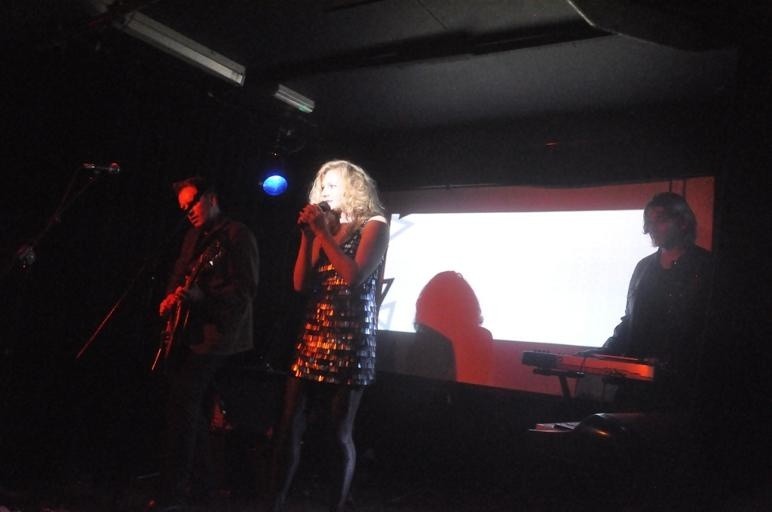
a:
[522,350,658,383]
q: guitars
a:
[158,244,223,361]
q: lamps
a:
[111,11,315,115]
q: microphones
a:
[298,201,330,225]
[83,161,122,174]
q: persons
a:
[568,188,728,413]
[268,159,391,512]
[139,176,264,510]
